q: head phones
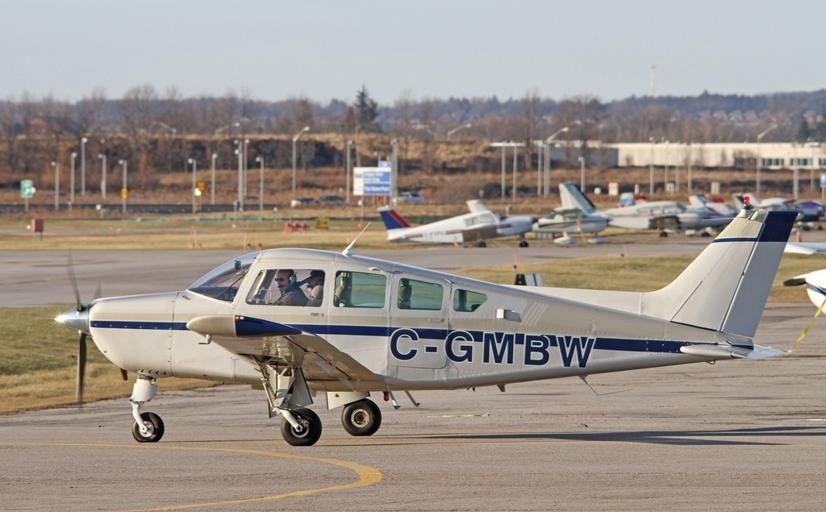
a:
[288,270,296,285]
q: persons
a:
[272,269,308,306]
[304,270,325,290]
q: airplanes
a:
[51,203,798,445]
[783,262,826,316]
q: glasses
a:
[275,278,284,281]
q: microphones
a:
[277,284,290,288]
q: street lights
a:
[649,137,696,195]
[69,152,78,201]
[756,124,780,193]
[97,155,107,196]
[291,127,311,193]
[188,123,266,212]
[501,126,588,197]
[117,159,128,213]
[412,117,473,218]
[80,138,88,194]
[345,135,399,205]
[50,161,59,209]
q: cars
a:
[291,197,320,208]
[321,195,343,204]
